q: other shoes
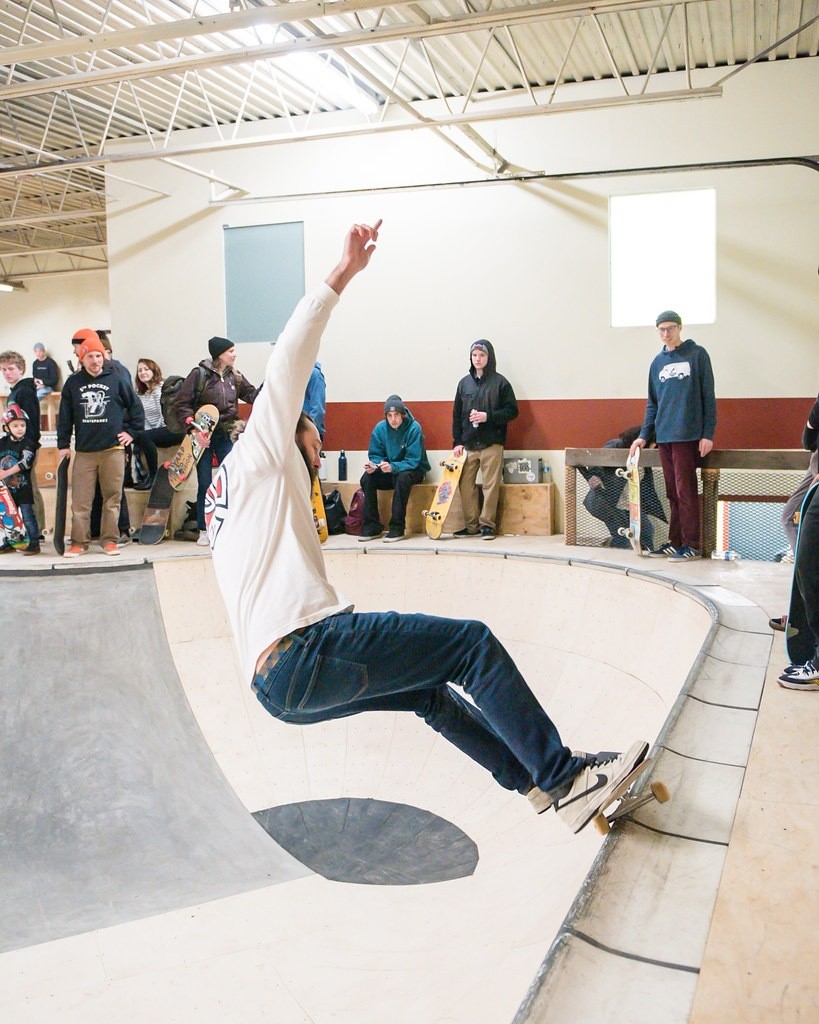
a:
[123,474,153,491]
[769,615,789,631]
[382,530,405,542]
[359,531,382,541]
[0,531,133,558]
[196,530,209,546]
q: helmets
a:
[1,407,30,432]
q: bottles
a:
[541,459,551,483]
[711,550,741,561]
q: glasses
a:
[656,324,678,333]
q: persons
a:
[769,393,819,691]
[205,218,649,834]
[172,336,257,546]
[358,394,431,542]
[71,328,132,549]
[134,358,185,490]
[100,336,134,389]
[452,339,518,539]
[630,311,717,562]
[0,407,41,556]
[0,349,45,541]
[579,425,668,552]
[58,328,145,557]
[301,362,326,440]
[32,343,60,400]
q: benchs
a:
[321,480,556,537]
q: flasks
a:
[339,449,346,480]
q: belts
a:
[251,627,305,695]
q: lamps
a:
[0,280,25,292]
[226,0,381,116]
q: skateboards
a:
[309,475,328,544]
[421,449,468,540]
[138,464,175,546]
[42,454,72,556]
[615,447,643,556]
[0,478,31,551]
[785,482,819,667]
[590,759,671,835]
[164,405,220,492]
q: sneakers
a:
[777,658,819,691]
[480,527,495,539]
[648,542,680,558]
[668,547,702,561]
[553,739,649,835]
[453,528,482,538]
[525,750,620,813]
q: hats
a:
[33,343,44,350]
[656,311,681,327]
[383,395,407,415]
[72,328,112,361]
[470,343,489,356]
[208,337,234,361]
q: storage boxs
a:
[502,457,544,484]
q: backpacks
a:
[324,490,347,535]
[345,489,366,534]
[160,366,241,436]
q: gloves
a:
[801,421,819,453]
